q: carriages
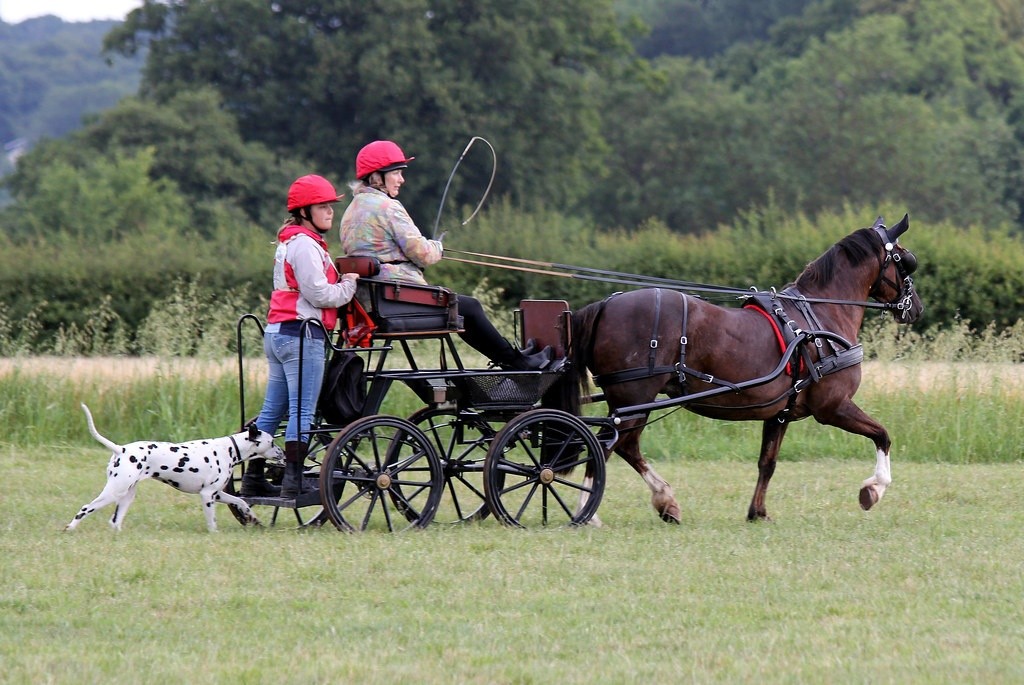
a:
[222,214,924,537]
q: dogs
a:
[63,402,285,533]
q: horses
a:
[560,213,924,529]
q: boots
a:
[279,441,316,498]
[241,441,283,498]
[491,337,539,367]
[492,338,556,382]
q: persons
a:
[239,173,360,499]
[339,140,555,391]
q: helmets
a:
[287,174,345,213]
[355,141,416,180]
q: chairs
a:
[337,273,465,372]
